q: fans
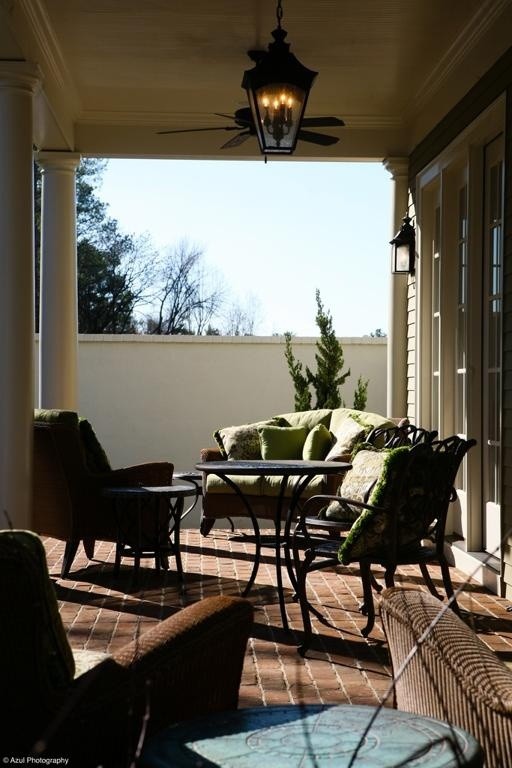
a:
[156,48,347,150]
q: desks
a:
[193,457,354,642]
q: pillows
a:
[318,441,394,522]
[215,417,287,459]
[321,412,373,462]
[302,422,334,460]
[335,445,416,566]
[258,425,308,459]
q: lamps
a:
[390,212,415,276]
[240,0,321,155]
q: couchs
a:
[30,409,174,579]
[198,408,409,540]
[0,527,254,766]
[377,587,512,765]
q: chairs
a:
[297,421,440,612]
[292,436,477,654]
